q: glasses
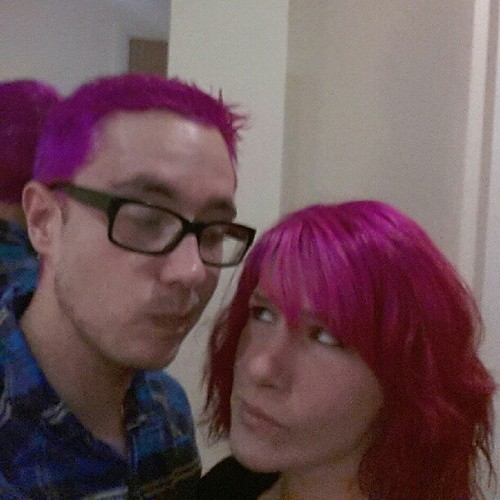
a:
[48,182,257,267]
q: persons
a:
[0,72,258,500]
[0,79,66,350]
[175,200,500,500]
